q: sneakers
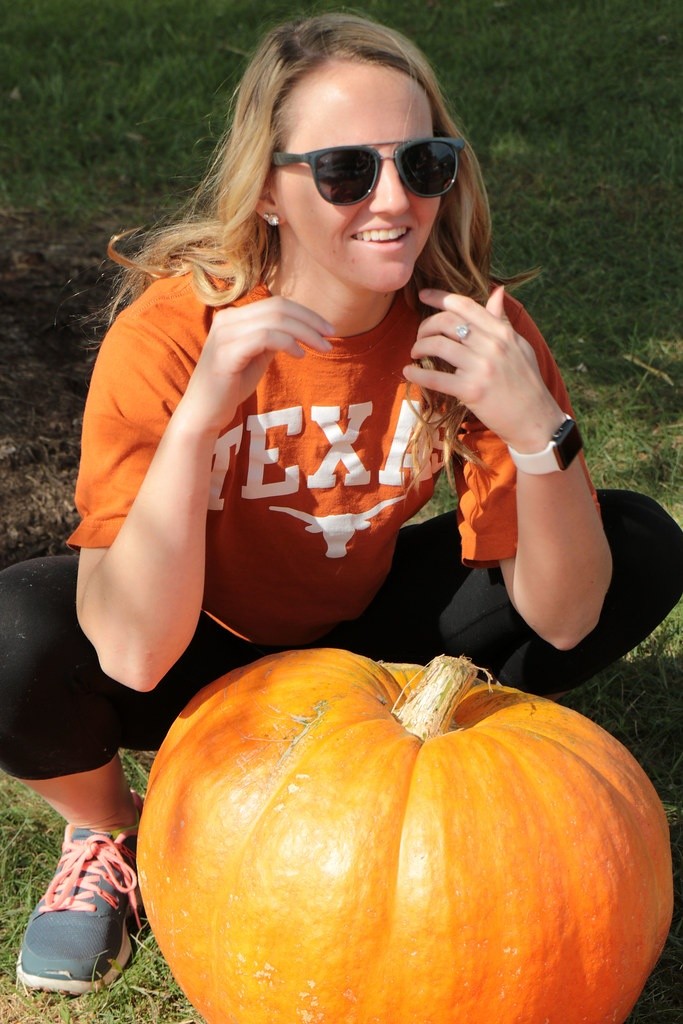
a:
[16,788,147,993]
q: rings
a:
[456,322,472,343]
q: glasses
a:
[270,129,465,206]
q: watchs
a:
[508,412,584,475]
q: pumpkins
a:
[136,647,673,1024]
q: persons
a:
[0,14,683,993]
